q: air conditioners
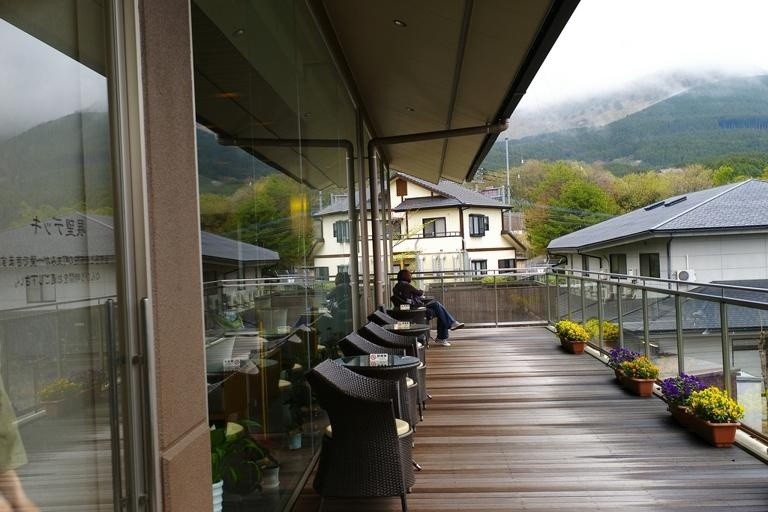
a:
[598,271,608,280]
[627,268,637,278]
[677,269,695,282]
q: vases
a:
[662,393,741,448]
[613,366,657,397]
[288,428,303,450]
[604,337,620,350]
[559,334,585,354]
[260,462,281,489]
[212,478,225,512]
[40,383,101,418]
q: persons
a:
[326,270,352,306]
[0,377,38,512]
[393,270,465,346]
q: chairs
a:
[303,294,435,511]
[205,292,353,416]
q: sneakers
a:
[450,320,464,330]
[435,339,451,347]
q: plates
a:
[224,330,261,361]
[205,337,235,374]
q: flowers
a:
[554,320,587,342]
[586,318,620,340]
[657,372,745,423]
[37,368,106,399]
[210,417,266,488]
[606,346,660,379]
